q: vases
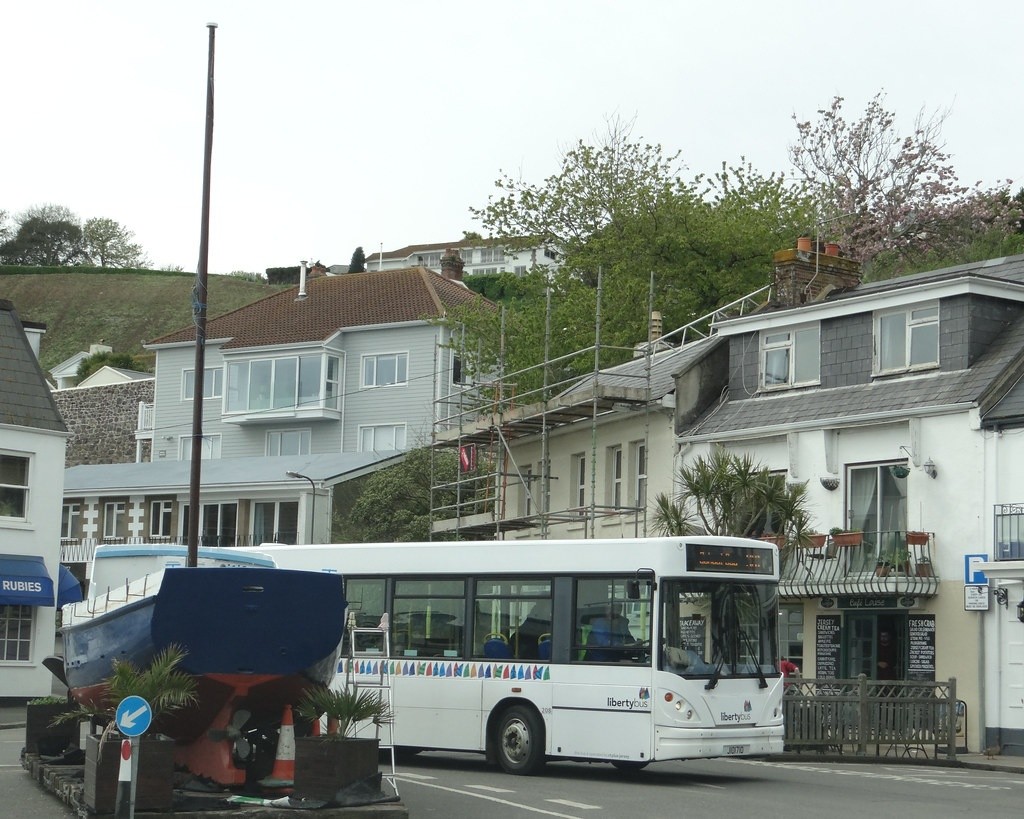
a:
[823,243,838,255]
[904,531,931,545]
[796,235,812,251]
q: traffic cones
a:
[253,701,296,788]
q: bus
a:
[89,536,786,779]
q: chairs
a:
[821,692,938,758]
[484,633,511,659]
[537,632,554,659]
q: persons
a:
[877,630,898,729]
[781,656,799,695]
[606,598,635,644]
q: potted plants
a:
[830,528,863,547]
[288,687,394,795]
[799,529,830,548]
[44,644,202,806]
[24,696,82,757]
[755,529,787,550]
[914,554,932,577]
[893,548,911,576]
[876,551,893,577]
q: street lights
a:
[286,470,316,545]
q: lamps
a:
[1016,600,1024,623]
[975,584,1009,609]
[923,457,938,478]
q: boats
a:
[53,541,352,789]
